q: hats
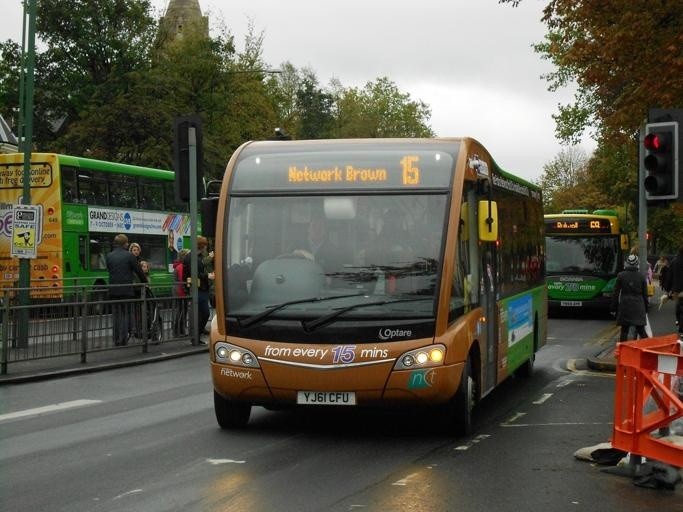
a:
[624,255,640,267]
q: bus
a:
[0,152,216,315]
[543,209,631,319]
[209,136,548,432]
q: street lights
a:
[210,68,282,124]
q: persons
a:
[610,244,682,341]
[172,249,191,338]
[106,234,149,345]
[129,243,144,292]
[140,260,150,280]
[183,235,214,346]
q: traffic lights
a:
[644,131,669,193]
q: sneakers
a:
[200,340,207,345]
